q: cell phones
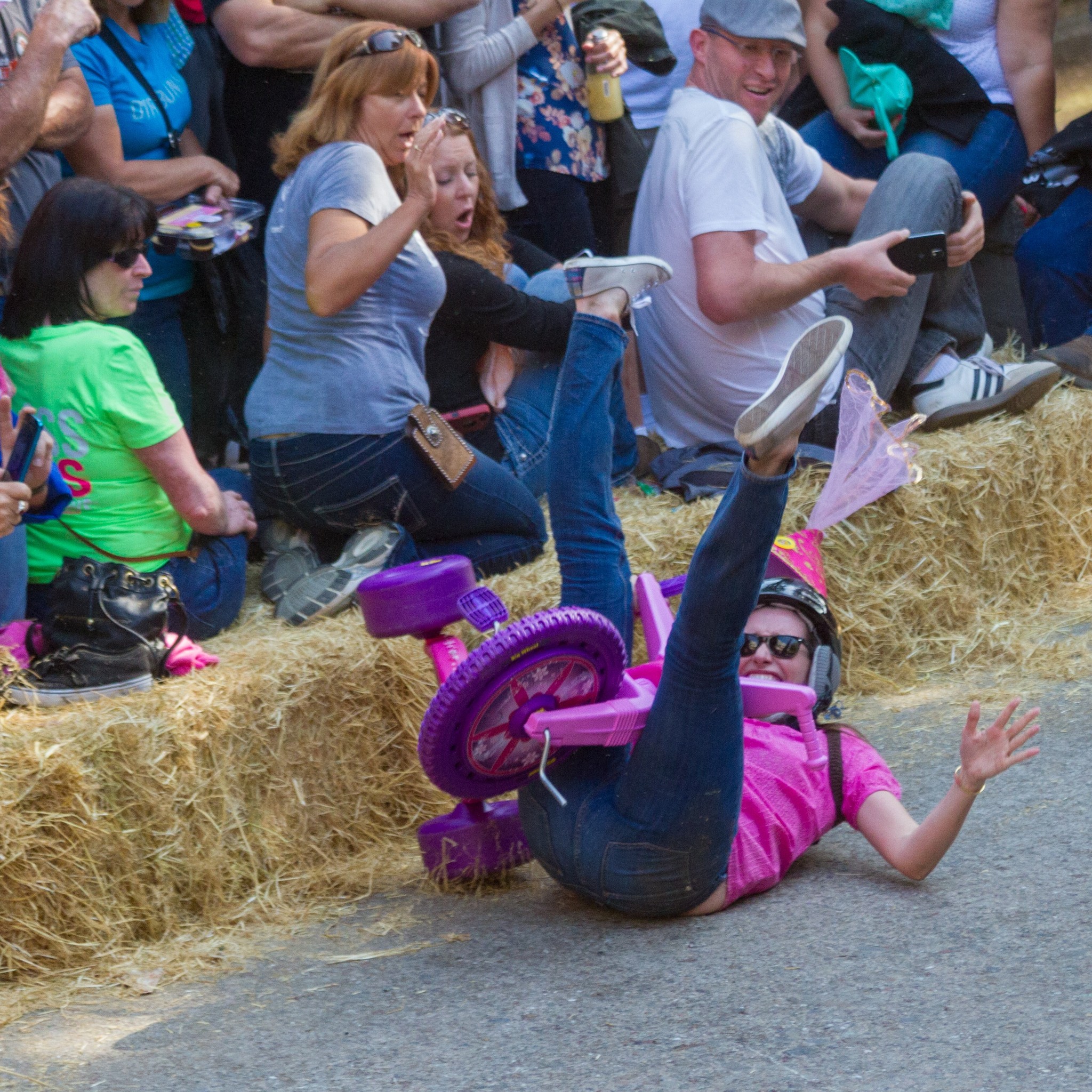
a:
[886,230,947,275]
[0,413,43,482]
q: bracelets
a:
[26,482,49,497]
[955,765,986,796]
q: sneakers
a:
[273,521,403,629]
[562,254,674,337]
[256,511,317,608]
[3,643,154,708]
[908,353,1061,433]
[732,314,854,461]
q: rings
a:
[16,499,27,516]
[414,144,423,154]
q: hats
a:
[699,0,808,48]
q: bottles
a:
[582,29,622,124]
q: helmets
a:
[755,574,840,726]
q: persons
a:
[382,104,664,499]
[505,256,1042,921]
[794,0,1092,390]
[0,390,74,542]
[2,175,261,640]
[626,0,1060,447]
[2,0,700,461]
[241,19,545,629]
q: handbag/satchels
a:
[202,248,252,338]
[839,47,913,162]
[24,553,187,682]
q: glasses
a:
[338,29,429,63]
[735,632,811,661]
[86,244,149,269]
[700,26,793,64]
[421,106,472,134]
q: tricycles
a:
[357,555,828,882]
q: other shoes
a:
[1022,334,1092,390]
[614,435,661,483]
